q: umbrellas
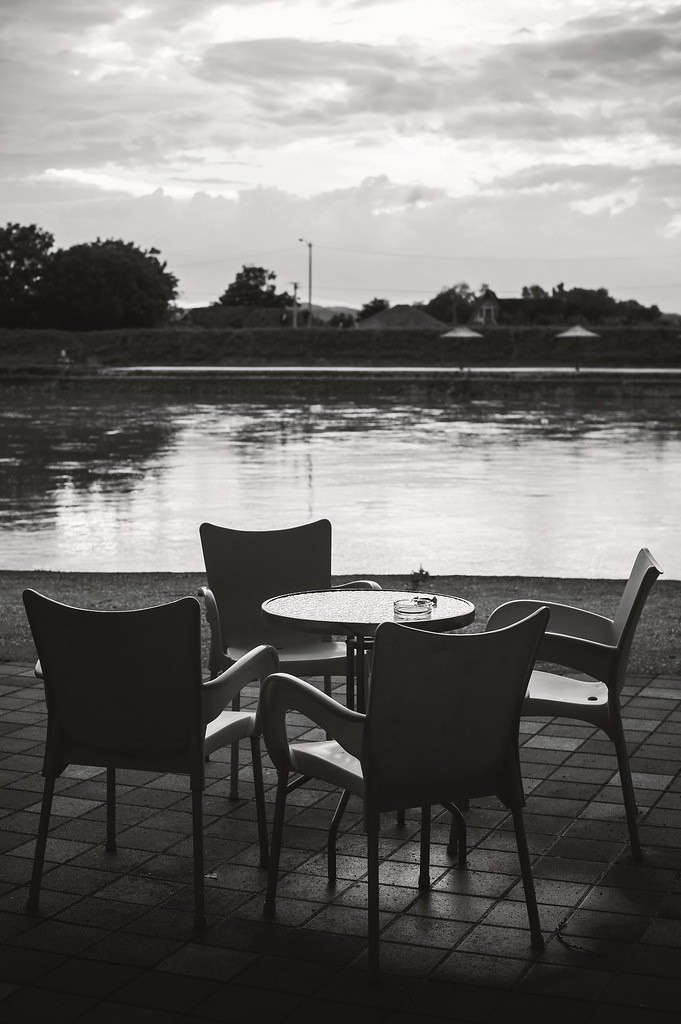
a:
[441,324,482,366]
[554,324,601,370]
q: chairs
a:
[259,606,552,969]
[20,588,281,930]
[197,520,383,799]
[454,549,663,863]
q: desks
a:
[260,588,477,885]
